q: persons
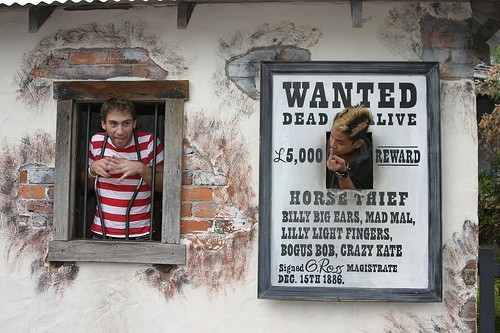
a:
[79,96,164,241]
[326,103,374,190]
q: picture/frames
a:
[257,59,444,304]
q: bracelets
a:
[88,164,97,179]
[334,162,351,181]
